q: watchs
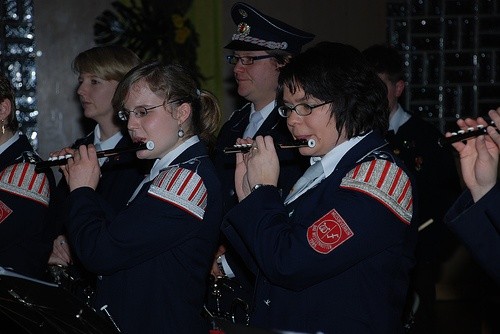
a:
[215,255,228,279]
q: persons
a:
[48,3,500,334]
[0,73,56,334]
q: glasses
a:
[277,100,333,118]
[227,55,272,65]
[118,99,182,120]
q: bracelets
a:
[251,184,262,193]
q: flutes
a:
[438,121,500,148]
[35,141,155,174]
[224,139,316,154]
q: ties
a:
[127,176,149,204]
[283,161,324,206]
[94,143,104,167]
[243,112,263,140]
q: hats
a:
[224,2,315,53]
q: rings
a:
[252,147,257,151]
[59,240,66,244]
